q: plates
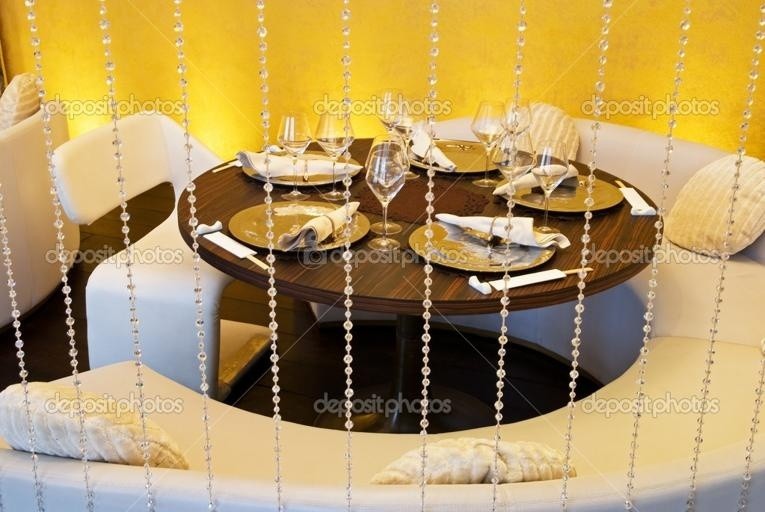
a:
[228,140,624,272]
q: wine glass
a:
[277,89,569,252]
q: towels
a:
[410,130,457,170]
[236,150,363,178]
[493,165,579,195]
[277,201,361,253]
[435,213,571,249]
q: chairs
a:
[53,110,273,401]
[1,102,80,326]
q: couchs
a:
[0,116,765,511]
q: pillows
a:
[1,382,190,471]
[1,74,40,131]
[663,153,765,257]
[513,101,580,160]
[370,436,577,485]
